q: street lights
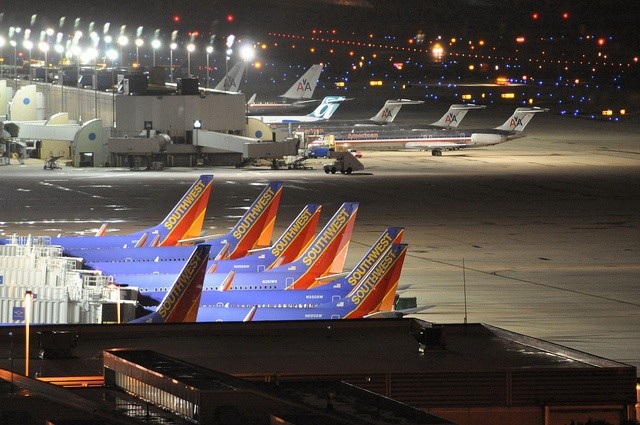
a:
[206,45,216,87]
[53,43,65,112]
[71,43,82,123]
[106,48,119,129]
[38,40,51,119]
[88,47,100,119]
[9,40,17,92]
[186,41,196,77]
[169,41,179,82]
[0,36,6,80]
[151,38,161,67]
[134,38,144,66]
[23,40,34,84]
[225,48,235,90]
[117,35,129,69]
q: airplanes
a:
[289,98,424,124]
[213,61,245,92]
[64,181,284,265]
[113,201,360,291]
[128,243,212,323]
[0,174,214,250]
[246,64,353,116]
[244,95,346,127]
[308,106,547,157]
[85,203,323,276]
[198,241,410,322]
[138,226,405,311]
[294,104,488,139]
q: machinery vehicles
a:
[323,156,353,174]
